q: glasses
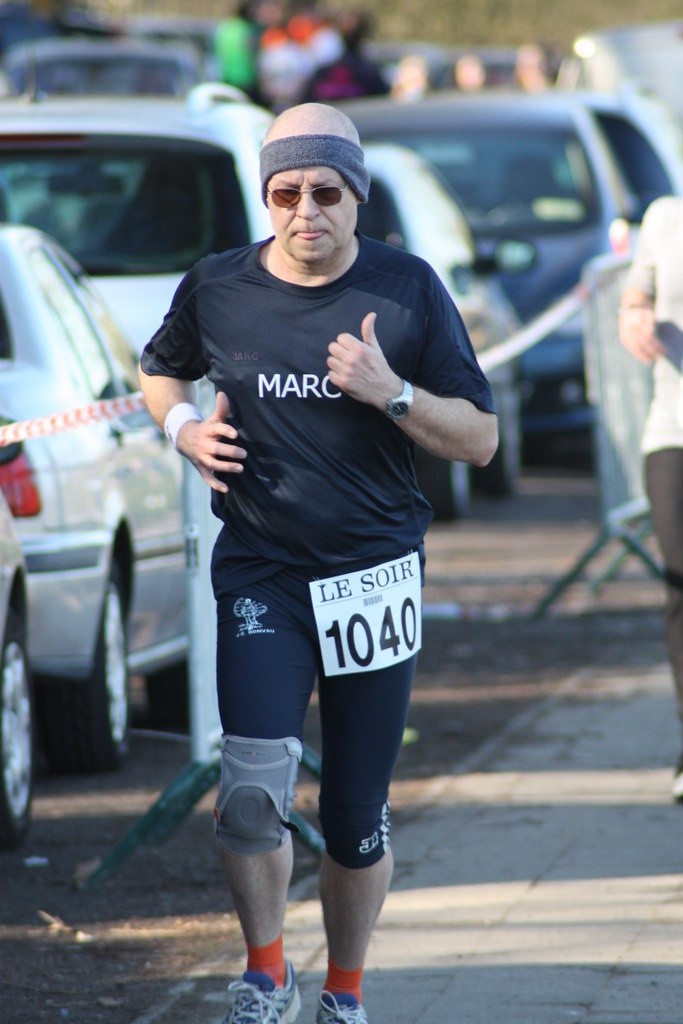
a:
[266,185,349,208]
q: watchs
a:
[386,379,414,420]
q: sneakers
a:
[316,989,368,1024]
[222,959,300,1024]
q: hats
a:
[259,103,370,209]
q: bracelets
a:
[164,403,204,456]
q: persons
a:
[136,102,502,1024]
[206,0,555,112]
[616,195,683,807]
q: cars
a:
[0,19,681,536]
[0,220,192,847]
